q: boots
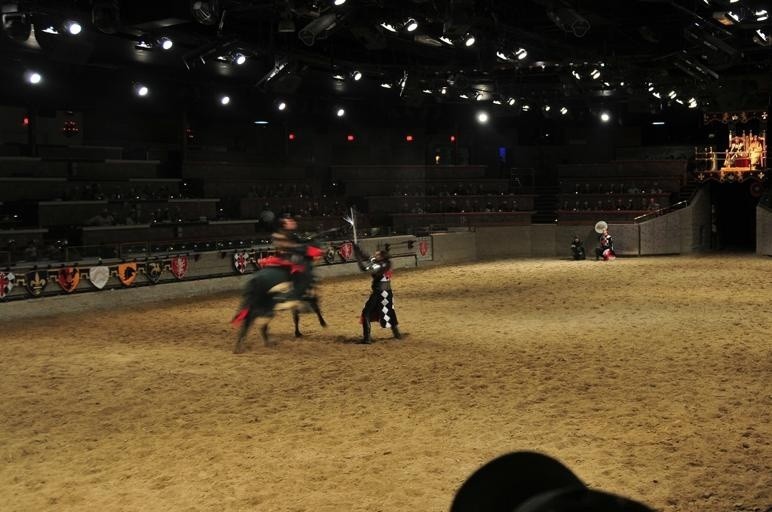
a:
[392,326,401,339]
[356,319,372,344]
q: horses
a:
[229,253,328,356]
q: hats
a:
[595,220,608,233]
[450,451,651,512]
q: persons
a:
[570,237,585,260]
[595,227,616,261]
[723,136,744,166]
[1,178,394,299]
[553,178,663,226]
[352,241,409,345]
[393,179,521,237]
[746,135,763,168]
